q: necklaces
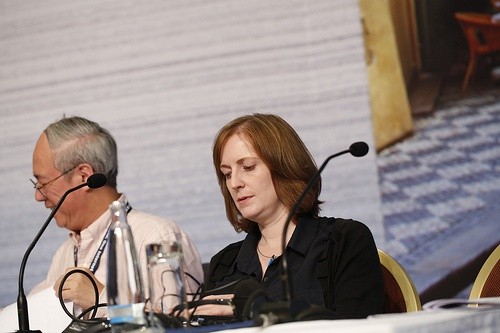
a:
[257,247,277,263]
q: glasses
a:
[29,162,95,198]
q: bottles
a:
[106,199,147,333]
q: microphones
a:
[253,142,370,324]
[13,174,107,333]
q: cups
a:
[146,240,190,328]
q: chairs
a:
[455,10,500,97]
[466,243,500,308]
[379,246,422,315]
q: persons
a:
[176,113,384,323]
[0,117,205,333]
